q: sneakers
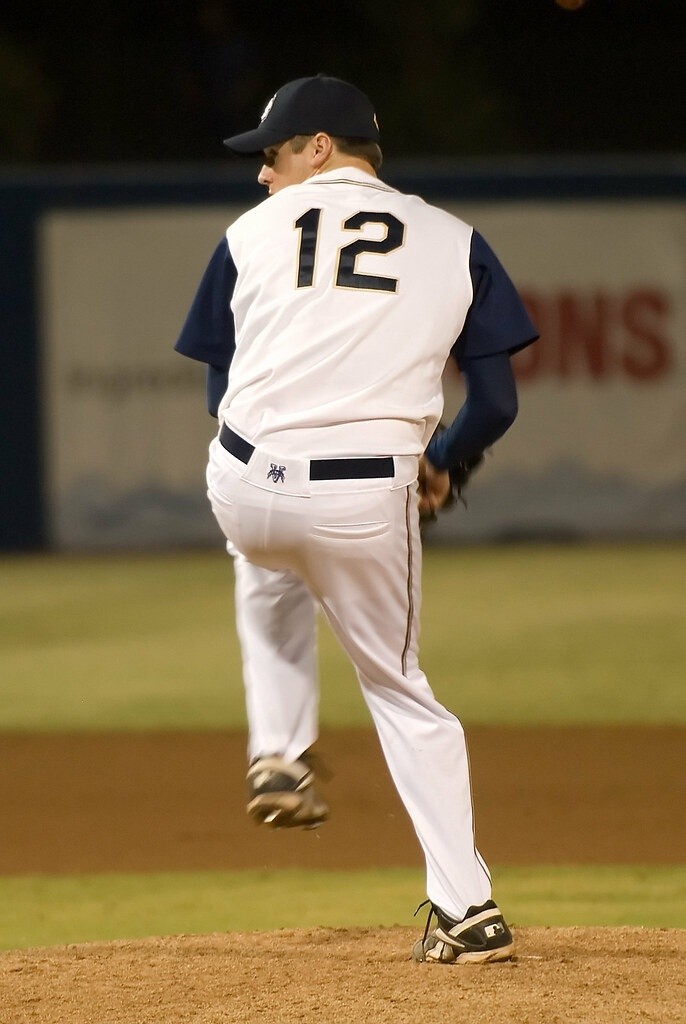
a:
[412,898,515,964]
[246,751,330,832]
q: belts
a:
[220,420,394,480]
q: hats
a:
[224,74,380,150]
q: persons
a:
[171,72,543,969]
